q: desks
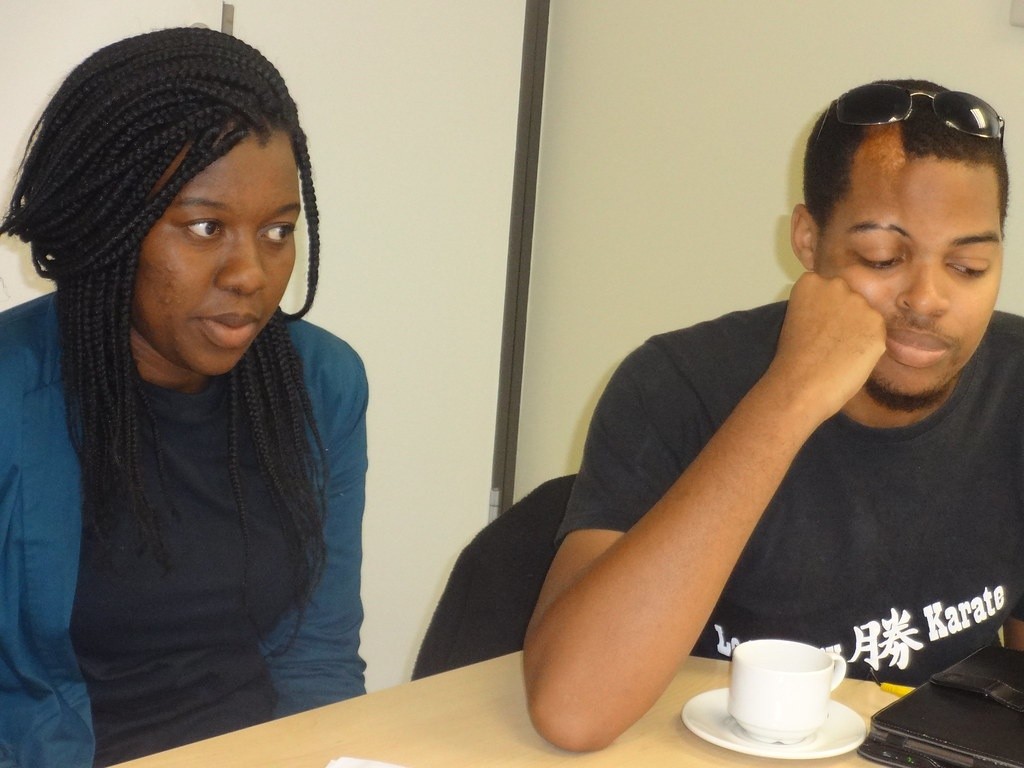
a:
[106,649,890,767]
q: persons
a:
[0,25,371,768]
[524,78,1024,753]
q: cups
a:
[729,639,847,745]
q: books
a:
[858,641,1024,768]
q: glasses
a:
[812,83,1006,153]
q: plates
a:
[681,687,867,760]
